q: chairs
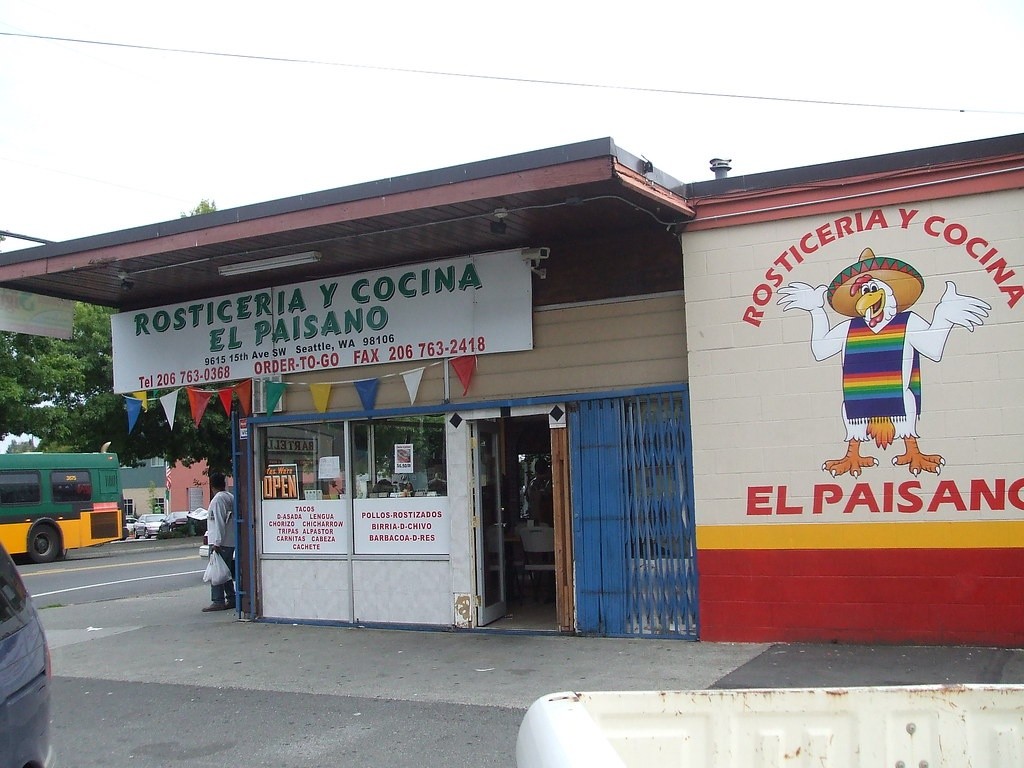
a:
[484,523,504,572]
[520,526,556,606]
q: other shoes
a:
[225,601,236,610]
[202,604,226,612]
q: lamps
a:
[115,272,134,292]
[217,251,322,277]
[490,207,510,234]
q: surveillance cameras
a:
[522,246,550,260]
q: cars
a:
[124,517,137,532]
[132,513,167,540]
[198,530,236,562]
[0,544,57,768]
[158,510,193,534]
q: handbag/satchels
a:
[202,551,233,586]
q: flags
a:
[166,461,172,492]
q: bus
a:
[0,440,129,564]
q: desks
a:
[501,537,529,543]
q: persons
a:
[202,473,236,612]
